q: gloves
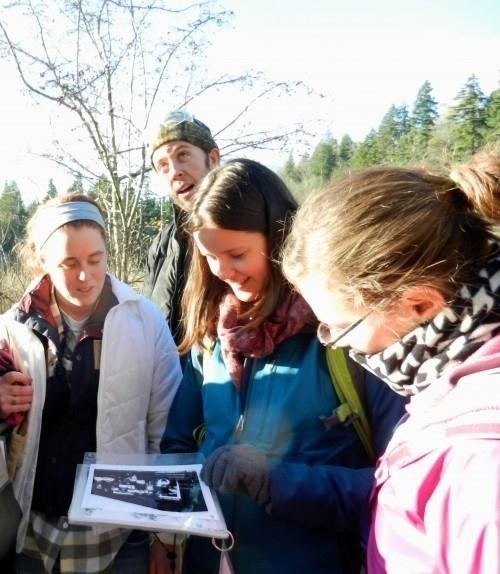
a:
[199,443,271,505]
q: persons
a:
[0,189,182,574]
[276,146,499,573]
[154,157,408,573]
[140,116,221,368]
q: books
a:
[67,450,235,553]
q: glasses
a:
[161,110,202,129]
[316,296,388,352]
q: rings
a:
[10,395,17,405]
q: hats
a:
[150,115,220,173]
